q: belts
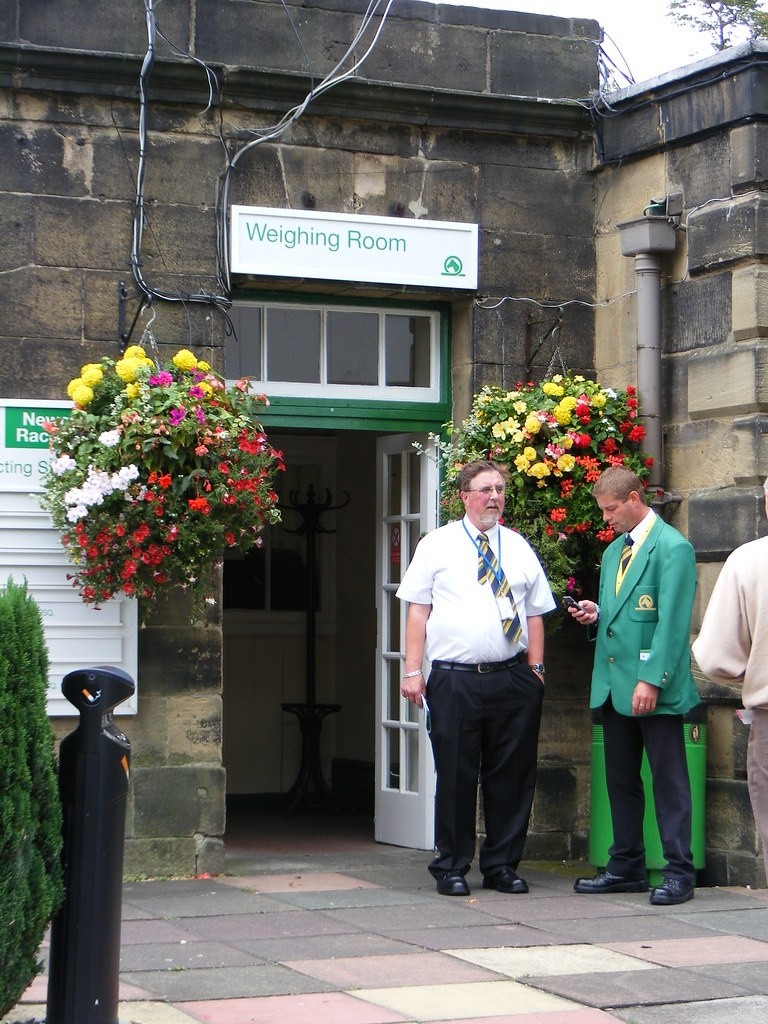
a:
[432,652,525,674]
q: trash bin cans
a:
[584,697,709,884]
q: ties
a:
[477,533,522,644]
[622,535,634,575]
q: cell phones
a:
[564,595,586,615]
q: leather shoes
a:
[483,868,528,893]
[574,872,649,893]
[437,876,471,895]
[649,878,695,904]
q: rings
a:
[401,692,404,694]
[405,696,410,698]
[640,704,645,707]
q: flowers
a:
[411,373,665,645]
[42,344,287,646]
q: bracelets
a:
[595,603,600,625]
[403,669,423,678]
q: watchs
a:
[529,663,545,675]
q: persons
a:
[691,477,768,886]
[394,460,558,896]
[567,466,702,905]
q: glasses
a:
[465,485,504,495]
